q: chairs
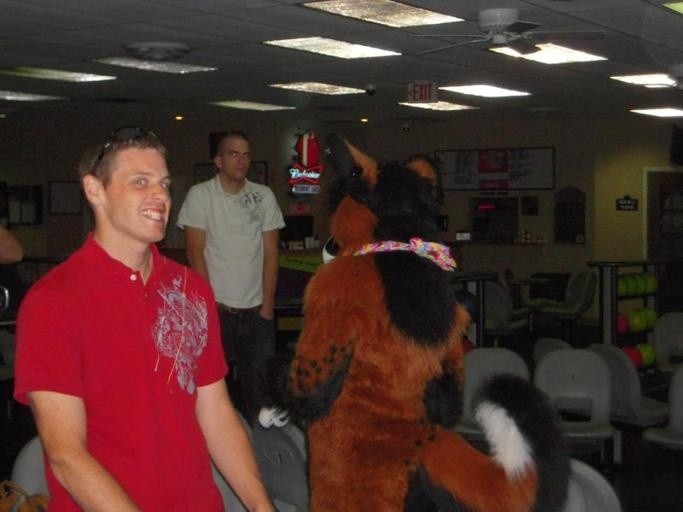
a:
[529,268,597,323]
[13,313,683,512]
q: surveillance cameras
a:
[366,85,376,96]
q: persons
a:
[175,129,288,427]
[1,223,25,265]
[11,123,276,512]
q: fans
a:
[409,9,607,55]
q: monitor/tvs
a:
[288,166,323,196]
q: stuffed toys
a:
[254,128,575,512]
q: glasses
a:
[94,125,167,174]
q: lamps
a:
[126,42,190,61]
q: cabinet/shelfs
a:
[587,257,673,394]
